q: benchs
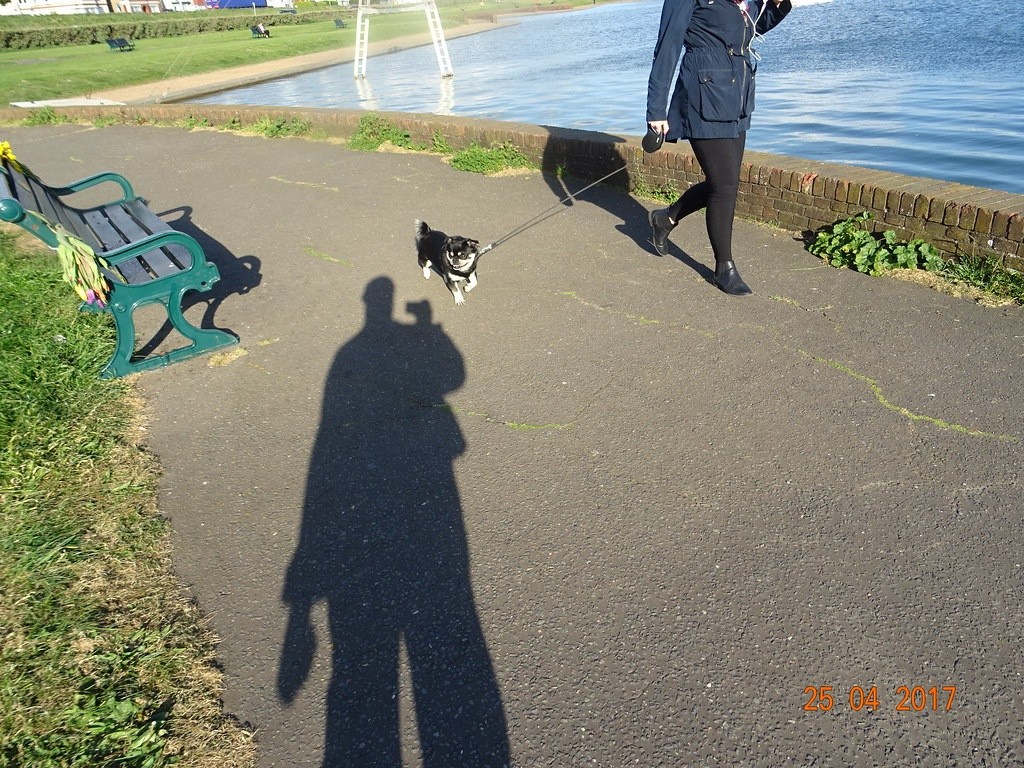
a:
[333,19,348,29]
[250,26,269,38]
[106,38,135,52]
[0,154,241,381]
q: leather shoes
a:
[712,259,753,296]
[648,206,678,257]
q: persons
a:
[645,0,793,295]
[258,23,269,37]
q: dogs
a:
[413,219,480,306]
